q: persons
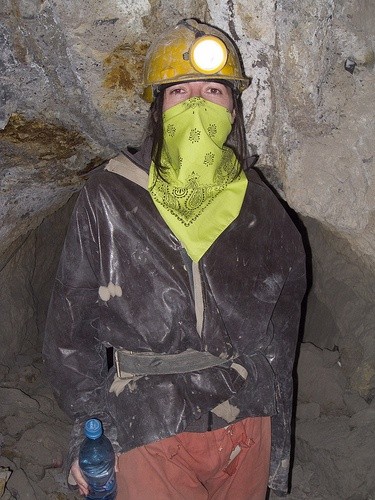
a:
[42,16,312,500]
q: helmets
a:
[139,19,251,105]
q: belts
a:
[109,346,228,376]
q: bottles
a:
[80,419,117,500]
